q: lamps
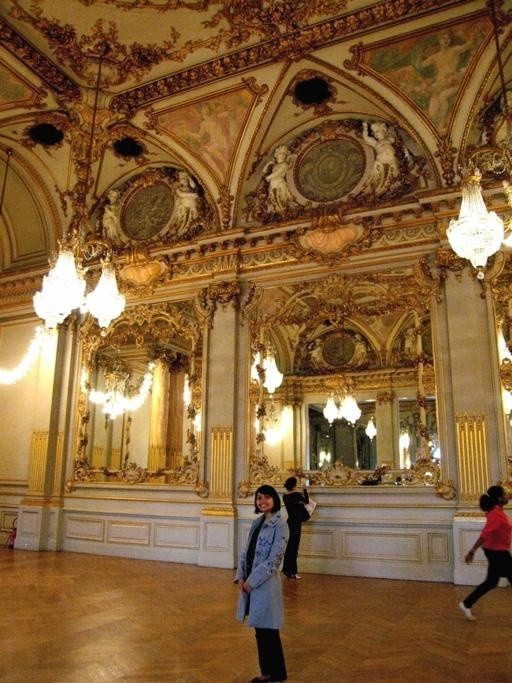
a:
[30,208,126,339]
[321,389,410,450]
[78,359,202,435]
[442,164,504,281]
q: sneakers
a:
[458,602,475,621]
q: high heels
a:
[252,675,286,683]
[283,571,301,579]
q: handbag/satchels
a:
[300,499,317,520]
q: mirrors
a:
[75,343,204,489]
[301,367,440,482]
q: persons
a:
[103,190,124,236]
[175,172,200,211]
[234,485,289,683]
[363,122,399,177]
[263,146,290,198]
[458,486,512,621]
[283,477,309,579]
